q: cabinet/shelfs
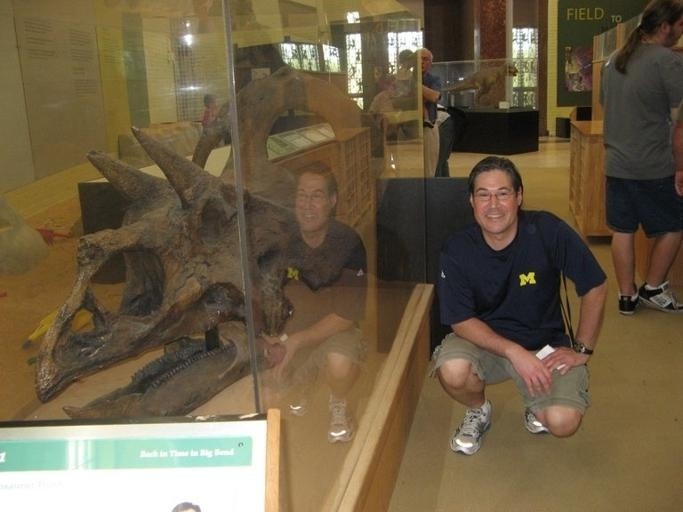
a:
[417,53,550,159]
[566,110,610,248]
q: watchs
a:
[572,341,593,354]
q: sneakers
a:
[524,410,549,434]
[449,399,492,455]
[619,280,683,315]
[328,396,354,442]
[290,396,310,415]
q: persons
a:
[598,0,682,315]
[201,93,216,133]
[422,156,607,457]
[258,160,368,445]
[363,82,395,112]
[434,104,457,178]
[671,99,682,197]
[388,50,414,91]
[397,48,441,178]
